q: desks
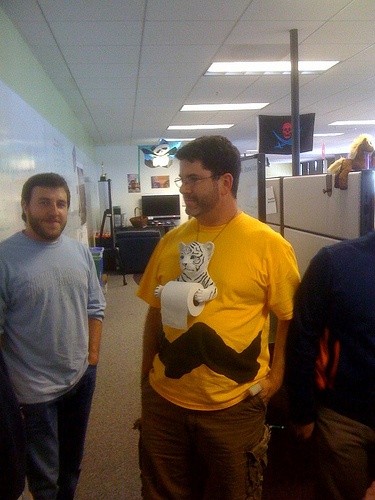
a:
[129,221,177,232]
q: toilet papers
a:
[161,281,203,328]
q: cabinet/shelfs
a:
[262,169,374,286]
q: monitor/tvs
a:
[143,194,180,219]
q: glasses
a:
[174,174,221,188]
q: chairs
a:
[115,230,161,285]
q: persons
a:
[285,221,374,496]
[2,172,107,495]
[134,135,300,499]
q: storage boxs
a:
[130,216,148,227]
